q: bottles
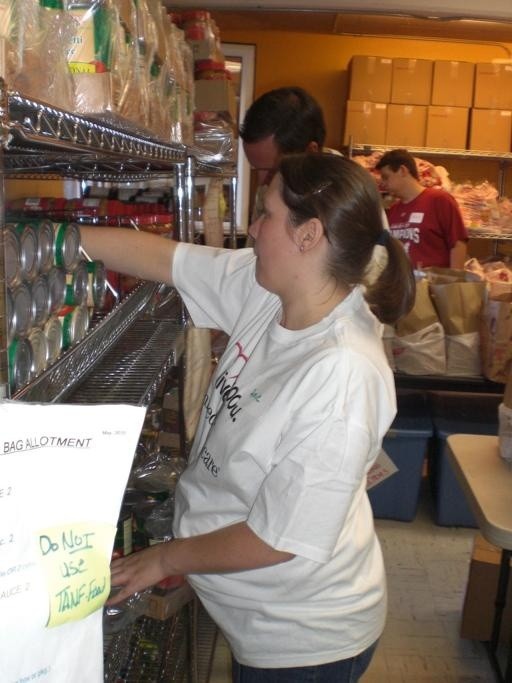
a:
[9,195,178,319]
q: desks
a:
[390,374,504,403]
[445,434,512,683]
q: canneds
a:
[0,216,107,396]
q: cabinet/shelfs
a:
[347,135,512,256]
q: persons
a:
[238,86,391,286]
[375,150,470,269]
[78,151,416,678]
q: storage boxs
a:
[342,54,512,153]
[459,533,512,645]
